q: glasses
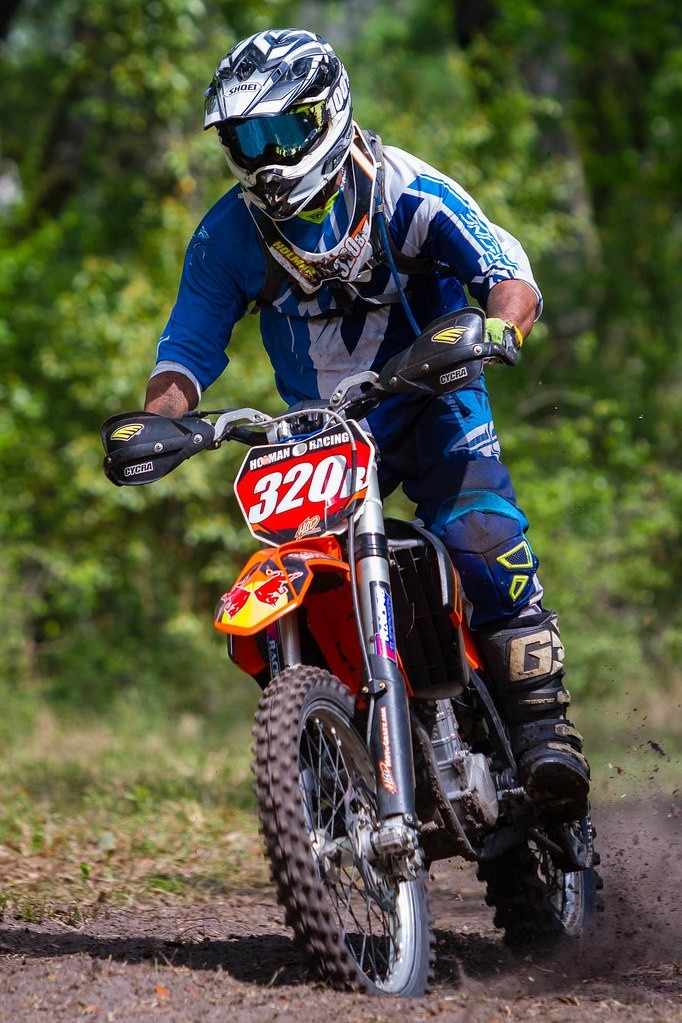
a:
[214,101,328,171]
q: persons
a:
[101,30,596,871]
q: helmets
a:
[204,28,355,222]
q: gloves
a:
[482,317,522,358]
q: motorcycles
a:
[101,308,603,1000]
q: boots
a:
[475,615,589,822]
